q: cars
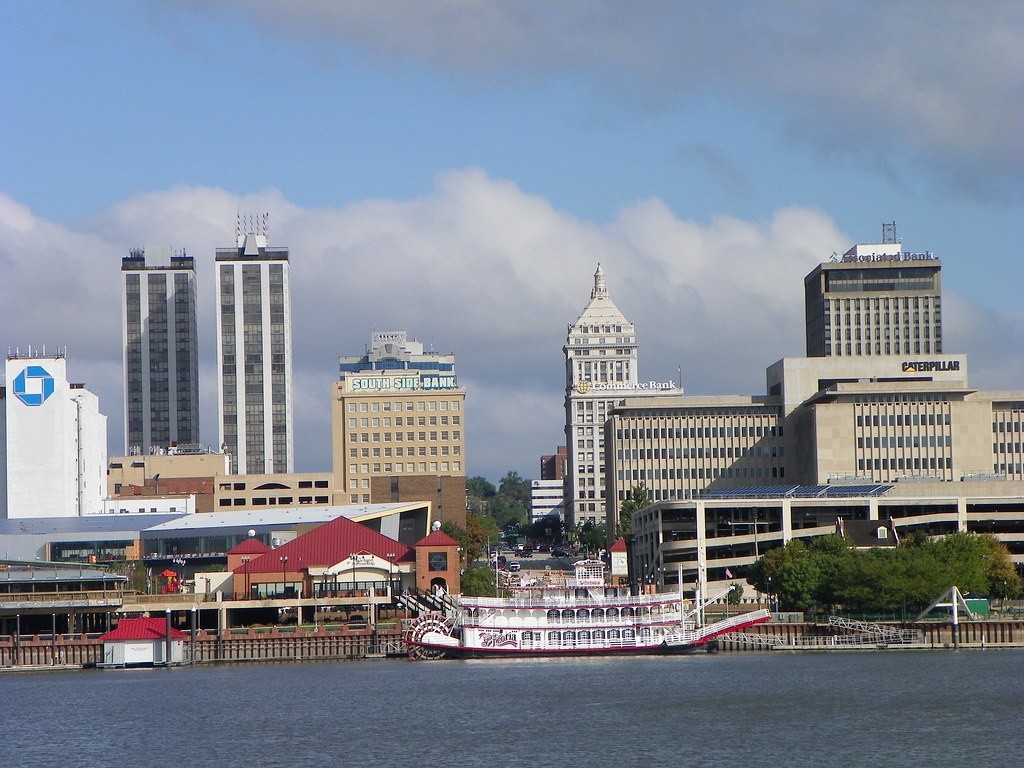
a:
[348,614,366,624]
[509,562,521,571]
[512,544,573,558]
[489,547,506,571]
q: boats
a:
[403,558,773,660]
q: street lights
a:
[350,553,358,596]
[242,556,249,600]
[1004,581,1007,610]
[387,553,395,597]
[178,560,186,579]
[280,556,288,599]
